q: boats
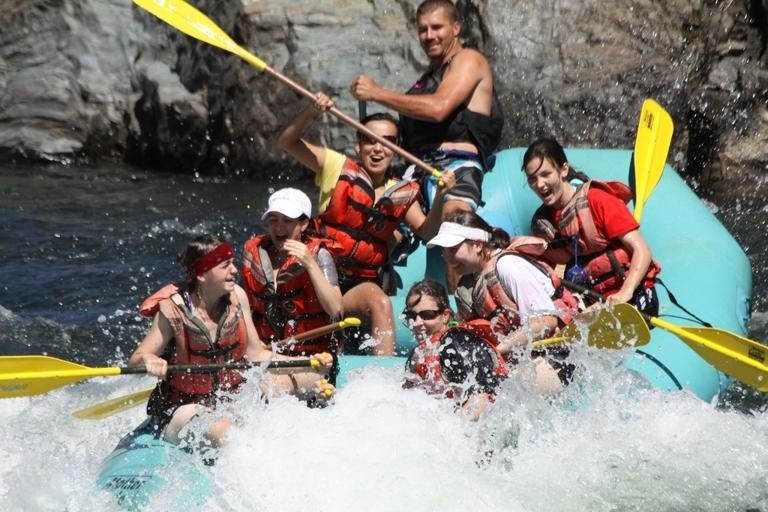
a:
[90,144,755,512]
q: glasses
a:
[359,133,400,147]
[402,305,444,321]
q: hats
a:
[424,219,494,250]
[260,186,314,221]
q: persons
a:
[130,235,333,461]
[522,138,658,331]
[277,91,456,296]
[242,187,396,391]
[350,0,493,293]
[405,280,509,421]
[426,210,581,396]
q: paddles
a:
[133,1,448,188]
[0,354,329,398]
[560,280,767,394]
[632,98,675,221]
[535,302,651,352]
[71,316,363,420]
